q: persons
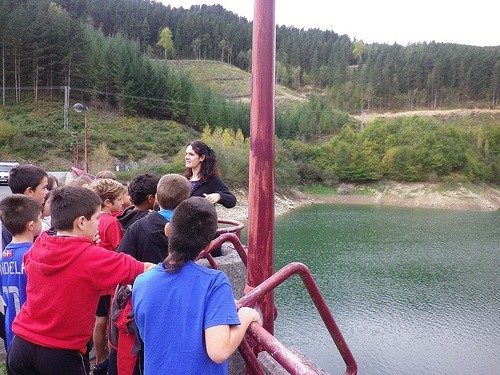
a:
[183,140,237,258]
[0,166,263,375]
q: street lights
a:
[71,132,79,169]
[73,103,88,173]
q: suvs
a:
[0,160,19,183]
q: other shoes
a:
[93,359,110,375]
